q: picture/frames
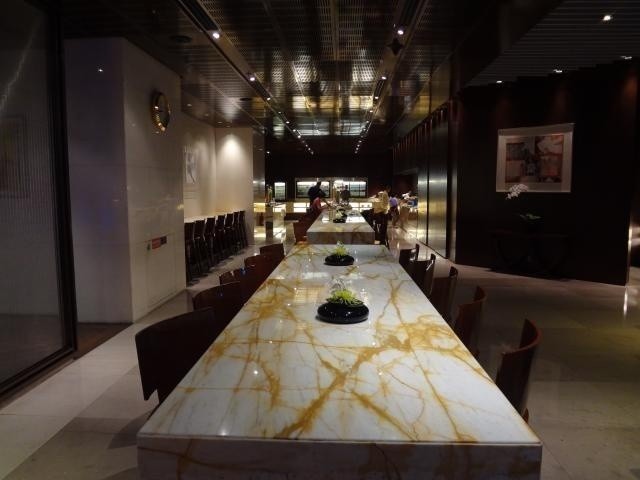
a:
[496,122,573,192]
[0,113,30,199]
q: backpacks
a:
[308,186,315,197]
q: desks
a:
[138,243,542,479]
[305,208,375,244]
[489,228,568,278]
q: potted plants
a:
[325,243,354,265]
[318,286,369,325]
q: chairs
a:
[181,210,250,282]
[220,268,252,308]
[259,243,285,265]
[359,208,390,249]
[134,306,216,401]
[494,319,542,423]
[292,206,321,245]
[193,282,243,336]
[454,285,487,363]
[431,266,459,329]
[398,243,419,270]
[244,255,276,291]
[410,254,436,299]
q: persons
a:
[340,184,351,202]
[401,190,412,200]
[307,181,332,210]
[368,184,400,244]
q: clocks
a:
[151,92,171,133]
